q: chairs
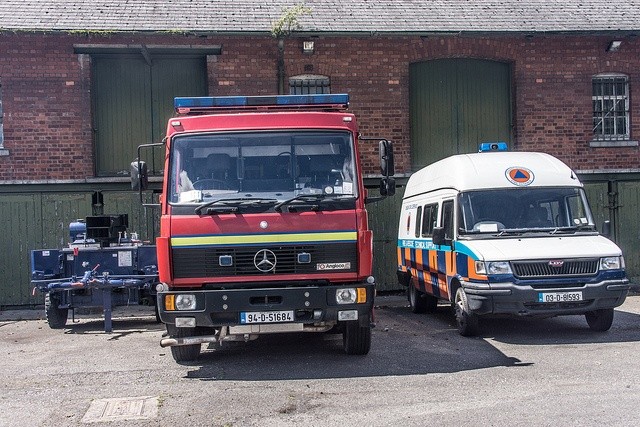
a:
[305,155,337,189]
[199,154,233,189]
[527,207,552,227]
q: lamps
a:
[606,40,623,52]
[303,41,314,54]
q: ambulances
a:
[397,143,629,337]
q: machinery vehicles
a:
[28,210,156,328]
[131,92,396,362]
